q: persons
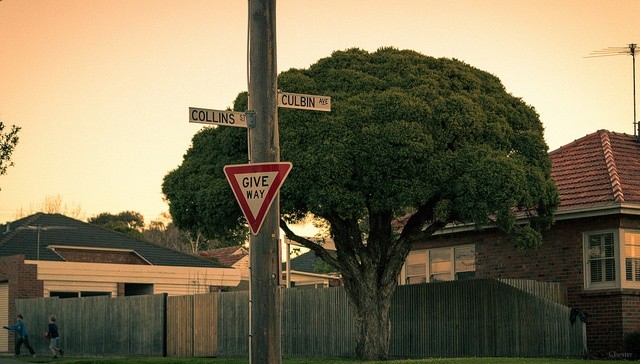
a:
[2,313,37,357]
[44,314,64,360]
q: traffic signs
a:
[276,92,331,113]
[223,161,293,236]
[188,107,246,128]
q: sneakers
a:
[60,349,63,355]
[31,353,36,358]
[52,355,57,360]
[13,355,19,358]
